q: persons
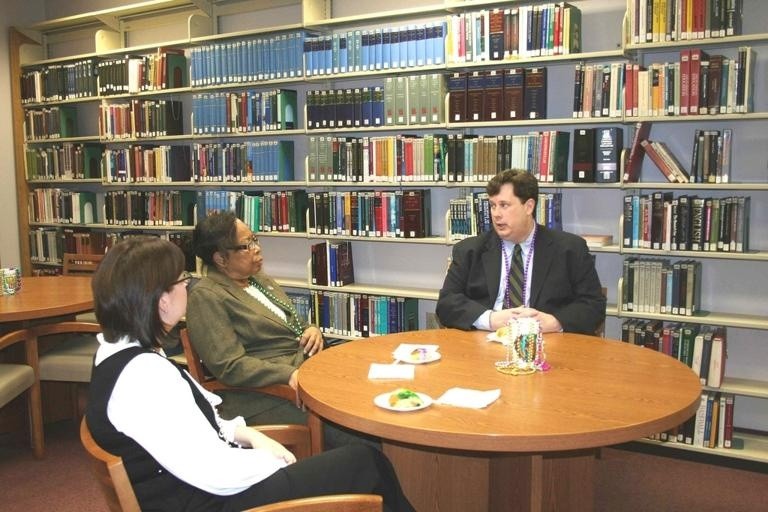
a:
[185,208,386,452]
[83,233,419,512]
[434,168,607,338]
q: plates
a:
[372,391,435,412]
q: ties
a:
[502,242,527,309]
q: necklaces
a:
[497,222,538,311]
[245,279,303,340]
[493,319,551,377]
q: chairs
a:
[593,286,608,461]
[79,412,385,510]
[180,326,322,460]
[0,327,47,462]
[28,320,104,450]
[62,252,104,277]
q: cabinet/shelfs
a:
[302,0,624,352]
[17,15,187,278]
[616,33,767,465]
[187,0,309,333]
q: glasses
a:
[213,235,259,253]
[167,269,194,288]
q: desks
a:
[296,327,703,512]
[0,277,95,326]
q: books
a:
[31,266,62,277]
[464,127,623,183]
[633,0,742,44]
[533,190,564,233]
[104,231,191,255]
[192,140,294,182]
[101,142,190,182]
[105,98,183,140]
[96,54,145,96]
[472,191,490,236]
[621,319,728,389]
[307,126,464,181]
[622,257,703,316]
[98,104,102,135]
[28,186,98,225]
[191,88,298,135]
[446,1,582,63]
[308,188,431,239]
[329,242,355,287]
[448,66,547,122]
[19,58,99,105]
[310,291,418,340]
[28,226,92,263]
[624,122,732,184]
[310,243,327,287]
[625,46,758,117]
[190,30,321,87]
[572,60,625,118]
[197,189,308,233]
[104,188,196,226]
[285,292,311,326]
[304,21,446,77]
[305,73,449,130]
[23,106,79,140]
[623,191,752,253]
[138,47,187,92]
[648,391,735,449]
[449,195,471,242]
[26,141,104,180]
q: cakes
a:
[389,390,422,408]
[411,347,428,361]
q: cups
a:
[513,319,540,369]
